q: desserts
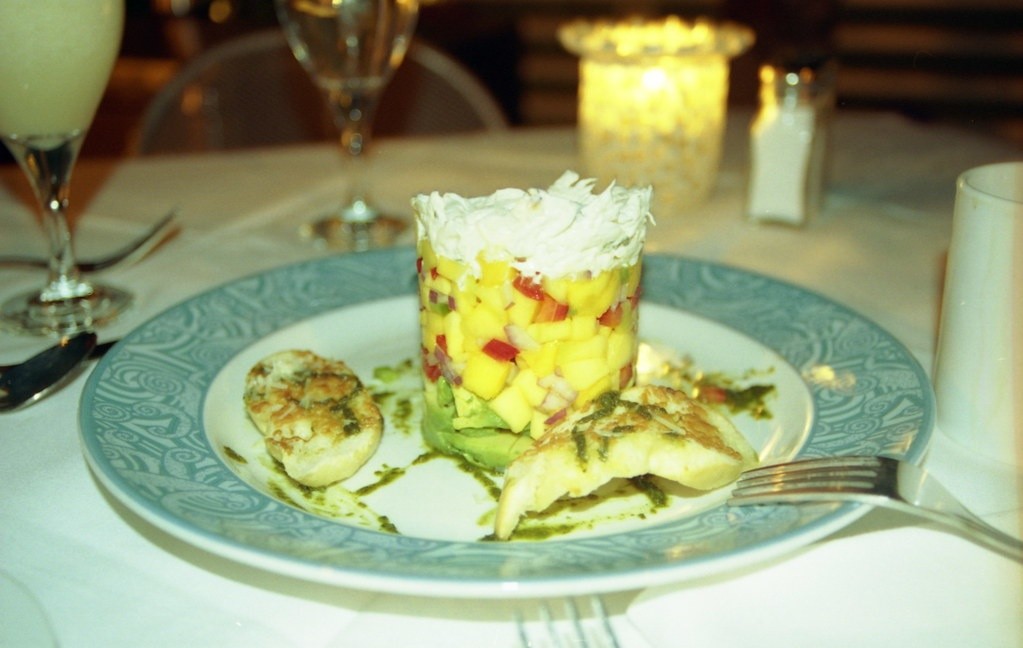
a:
[409,169,653,443]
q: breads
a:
[242,349,384,490]
[495,383,758,534]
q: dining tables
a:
[1,112,1022,648]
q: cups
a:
[934,162,1023,469]
[577,56,729,214]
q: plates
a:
[77,246,932,601]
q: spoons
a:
[0,330,99,413]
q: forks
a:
[0,210,180,277]
[726,457,1023,565]
[511,594,619,647]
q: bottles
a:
[746,64,829,229]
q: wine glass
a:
[0,0,136,340]
[273,0,421,251]
